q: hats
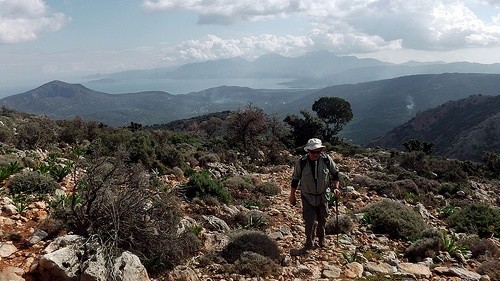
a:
[304,138,326,152]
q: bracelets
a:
[333,187,339,190]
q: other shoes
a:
[318,237,326,246]
[305,241,312,250]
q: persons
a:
[289,138,342,249]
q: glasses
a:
[309,150,322,154]
